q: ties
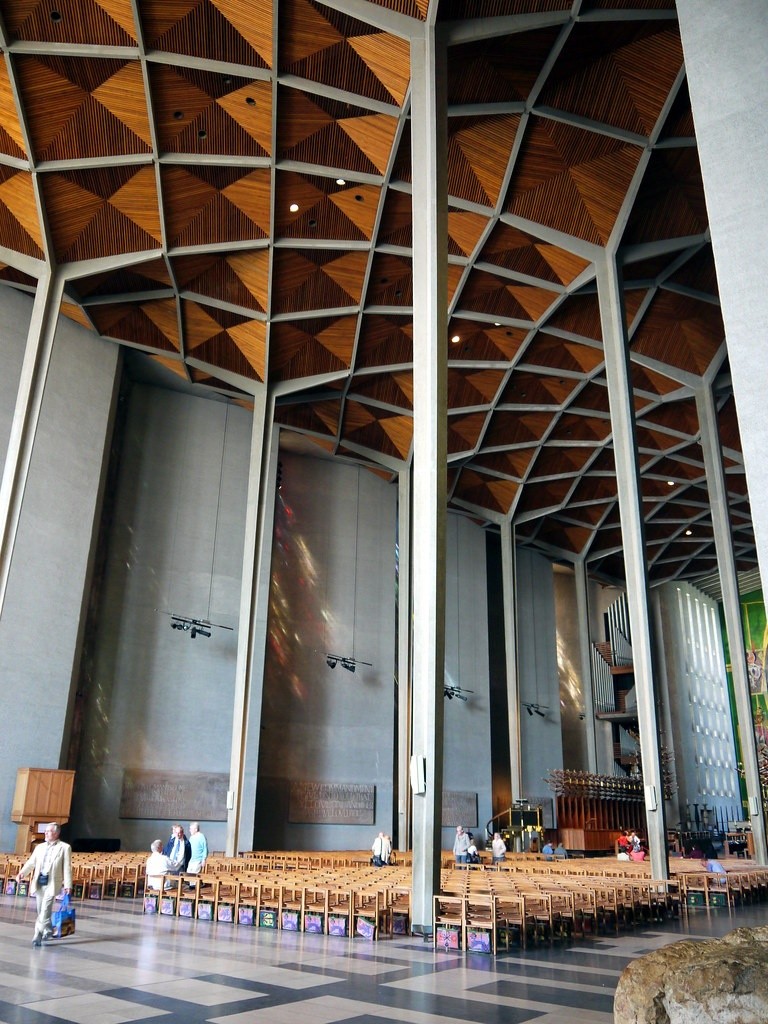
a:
[173,840,180,860]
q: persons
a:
[371,832,392,867]
[452,825,507,872]
[680,844,728,883]
[13,822,73,946]
[617,830,646,861]
[146,821,208,892]
[530,837,568,861]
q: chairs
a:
[0,847,768,960]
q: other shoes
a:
[32,930,53,946]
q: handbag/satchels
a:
[372,855,387,867]
[50,892,75,939]
[39,872,49,886]
